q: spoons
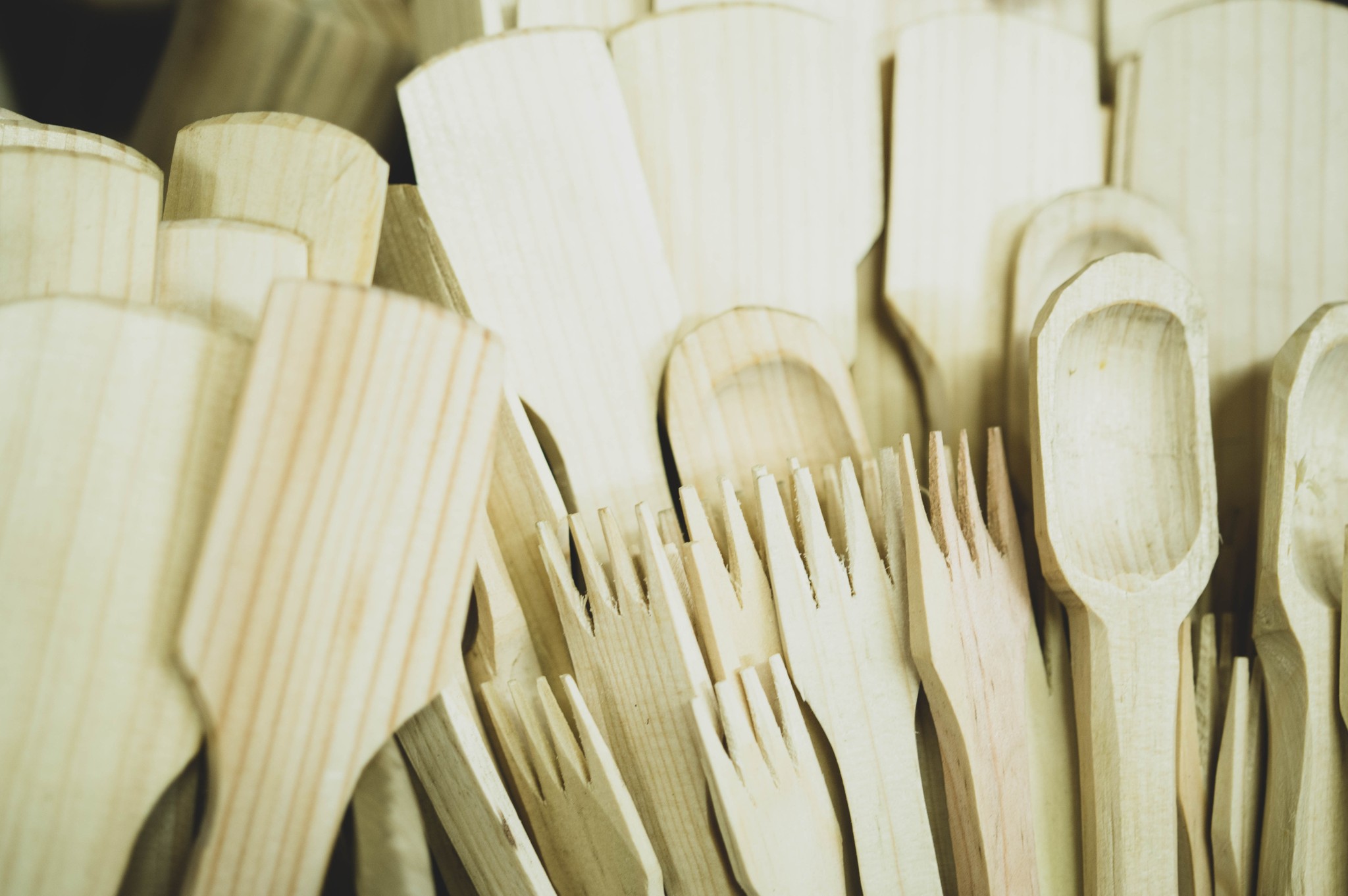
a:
[664,181,1348,896]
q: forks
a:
[490,429,1068,896]
